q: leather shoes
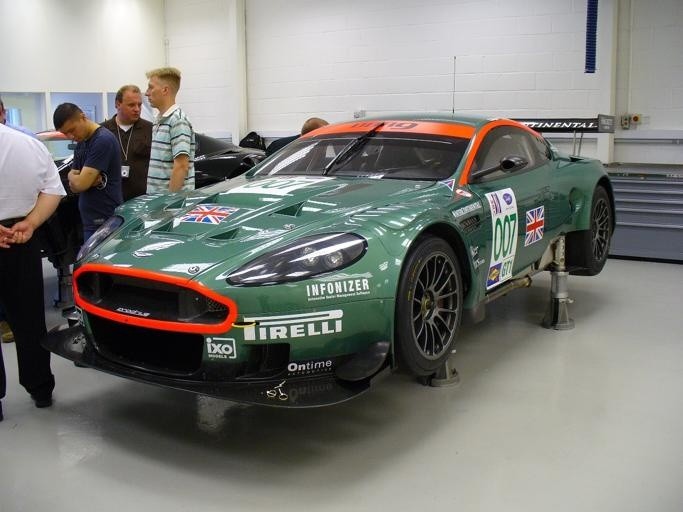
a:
[30,396,53,408]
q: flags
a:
[524,206,545,247]
[179,206,240,225]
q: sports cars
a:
[35,107,617,410]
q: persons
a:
[99,85,153,201]
[53,103,123,242]
[0,96,67,422]
[265,118,329,158]
[145,67,196,194]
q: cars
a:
[35,124,267,255]
